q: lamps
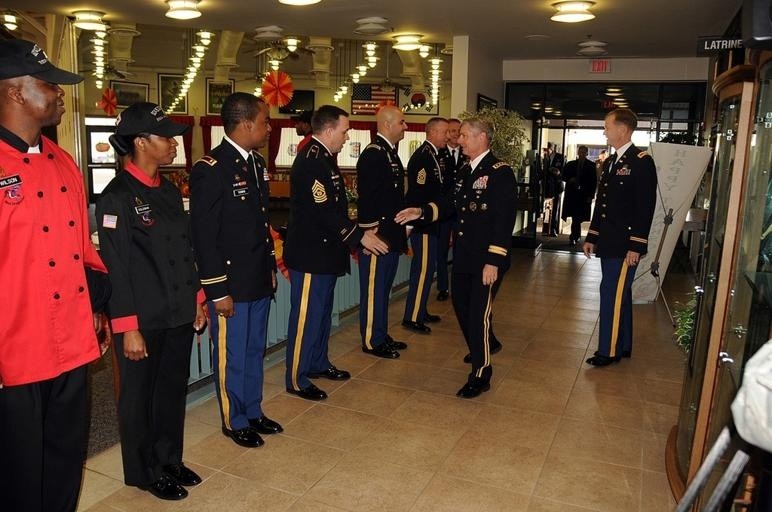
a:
[575,39,610,56]
[0,9,23,31]
[551,0,597,24]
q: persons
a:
[288,109,314,157]
[190,92,285,449]
[434,118,468,302]
[97,103,208,499]
[354,103,409,362]
[393,116,519,399]
[401,118,453,335]
[284,107,389,400]
[2,39,114,512]
[532,139,612,250]
[581,108,659,366]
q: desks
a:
[267,180,291,199]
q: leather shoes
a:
[456,376,491,398]
[222,425,264,447]
[593,350,631,358]
[361,341,400,359]
[424,313,441,323]
[585,354,620,367]
[248,414,284,434]
[148,470,188,500]
[569,236,576,245]
[401,320,431,334]
[308,364,351,380]
[383,334,408,350]
[168,462,202,486]
[286,382,327,400]
[436,288,449,301]
[464,335,502,363]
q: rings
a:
[219,311,224,316]
[632,259,636,263]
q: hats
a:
[290,110,311,122]
[0,36,85,85]
[115,102,191,138]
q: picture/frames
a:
[477,92,498,116]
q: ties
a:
[465,164,471,175]
[611,152,617,170]
[247,154,257,188]
[451,149,455,167]
